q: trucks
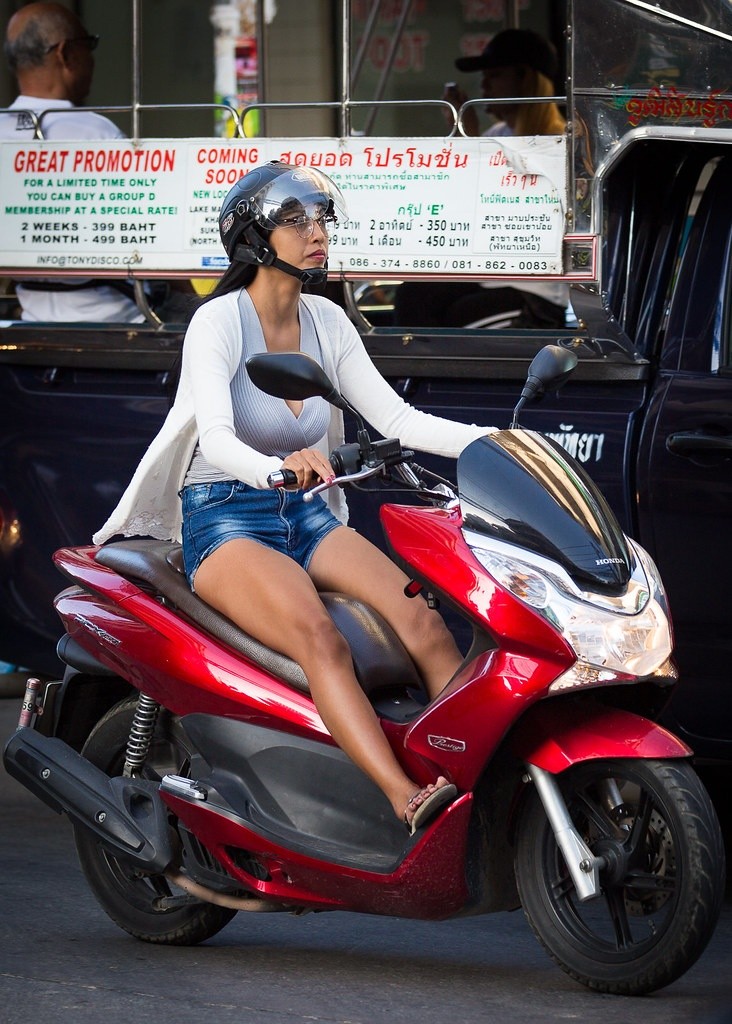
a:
[1,0,731,743]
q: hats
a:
[455,29,558,81]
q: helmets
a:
[218,161,335,263]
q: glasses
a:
[264,212,338,240]
[45,34,99,55]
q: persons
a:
[93,159,501,838]
[1,0,158,325]
[395,29,568,322]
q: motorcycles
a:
[0,345,729,995]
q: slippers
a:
[402,776,457,838]
